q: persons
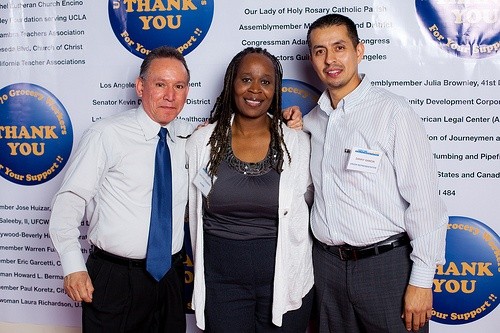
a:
[192,14,449,333]
[48,47,304,333]
[185,47,315,333]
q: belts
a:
[314,232,410,262]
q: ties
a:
[146,127,172,282]
